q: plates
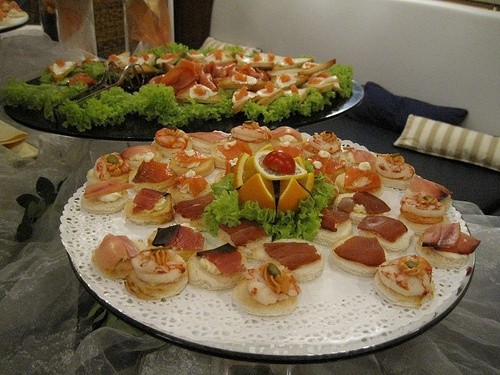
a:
[2,70,364,140]
[66,136,476,362]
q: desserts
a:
[80,121,482,310]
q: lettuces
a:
[0,43,355,131]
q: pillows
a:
[394,114,500,171]
[344,81,467,133]
